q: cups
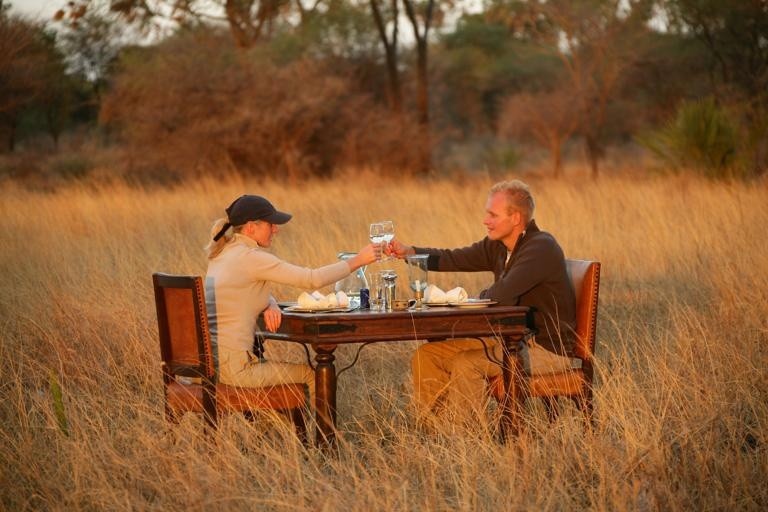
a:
[367,272,383,311]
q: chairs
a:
[152,272,318,462]
[486,260,603,444]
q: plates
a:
[284,304,358,313]
[423,300,497,308]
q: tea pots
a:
[335,250,370,310]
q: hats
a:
[225,195,292,227]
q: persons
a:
[384,178,577,435]
[174,191,383,455]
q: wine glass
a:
[406,254,431,311]
[369,221,395,262]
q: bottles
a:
[360,288,370,308]
[383,270,397,310]
